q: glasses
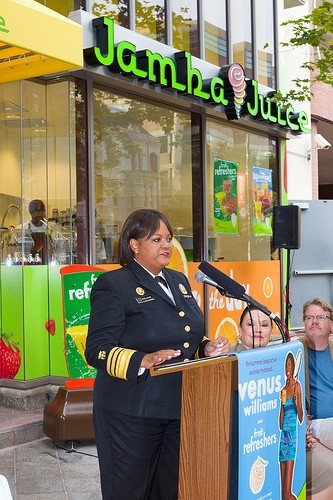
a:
[303,314,333,322]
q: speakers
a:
[272,205,301,249]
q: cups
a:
[254,182,270,219]
[215,180,236,217]
[281,389,286,405]
[67,325,93,368]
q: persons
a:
[228,305,273,353]
[15,200,77,259]
[84,209,230,500]
[300,298,333,452]
[279,352,303,500]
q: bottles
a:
[6,252,41,265]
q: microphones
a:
[195,271,225,289]
[202,262,245,298]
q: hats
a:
[29,200,45,217]
